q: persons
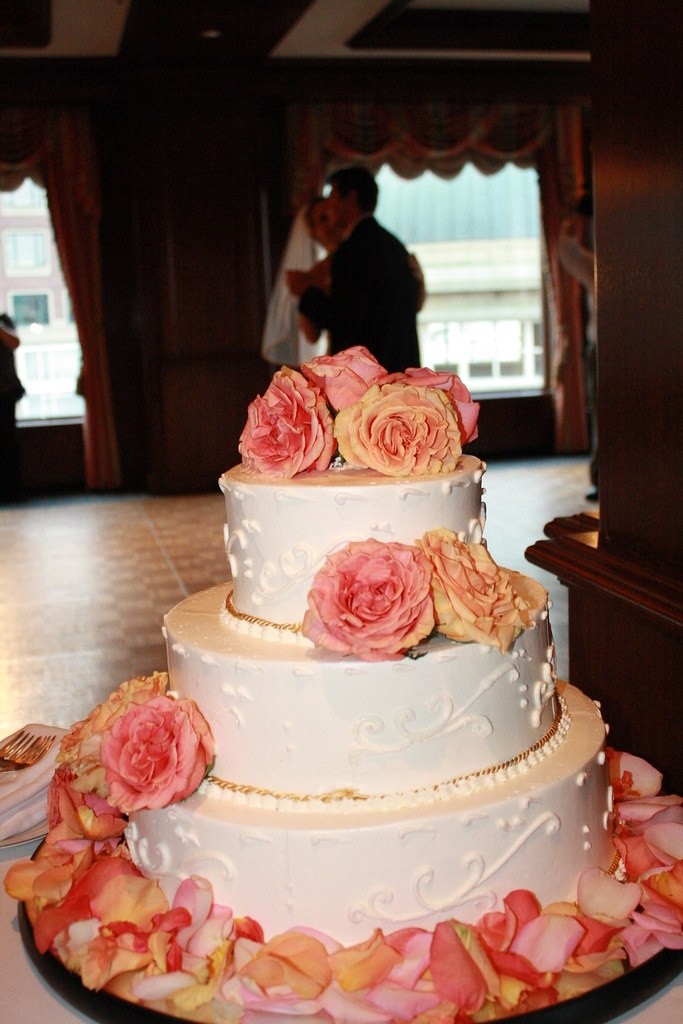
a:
[559,177,599,502]
[0,312,28,504]
[285,165,426,375]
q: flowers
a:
[90,343,551,815]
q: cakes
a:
[123,455,615,947]
